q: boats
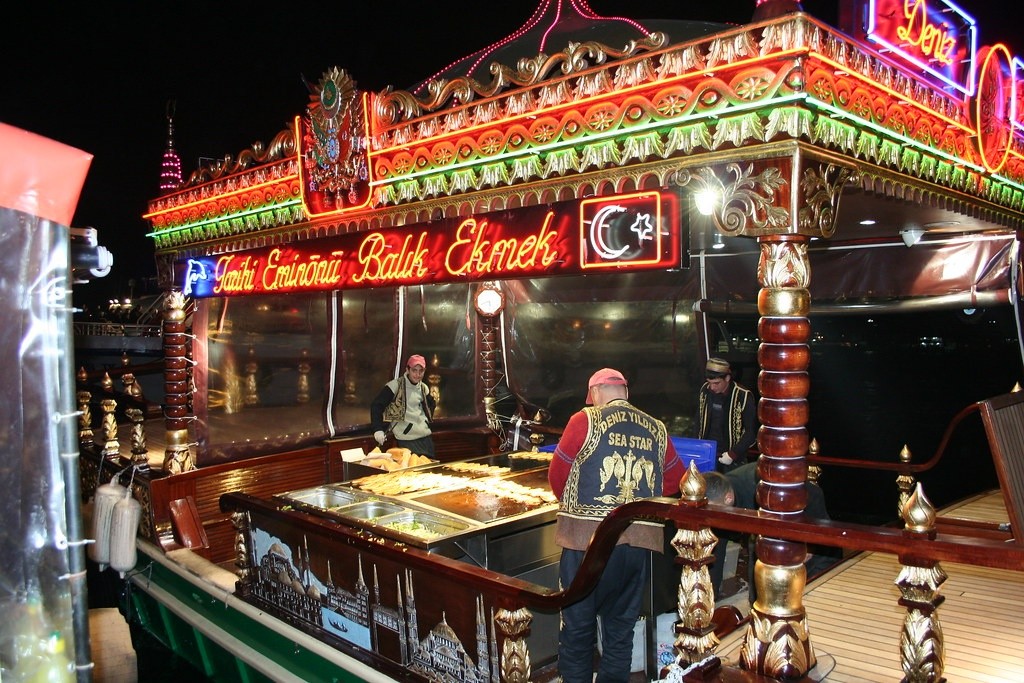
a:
[76,0,1024,683]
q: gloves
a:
[373,431,387,446]
[719,452,733,465]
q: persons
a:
[695,358,759,476]
[86,304,159,325]
[370,355,437,458]
[550,367,688,683]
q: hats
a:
[705,358,730,373]
[586,368,627,406]
[407,355,427,369]
